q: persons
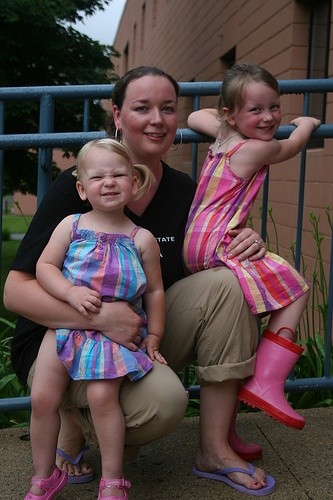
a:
[180,64,322,461]
[25,137,167,500]
[3,65,276,500]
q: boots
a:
[227,400,262,460]
[237,327,305,430]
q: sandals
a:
[97,473,130,500]
[23,465,67,500]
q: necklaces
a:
[216,132,241,151]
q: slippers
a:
[192,462,275,496]
[55,449,96,483]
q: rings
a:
[254,240,261,248]
